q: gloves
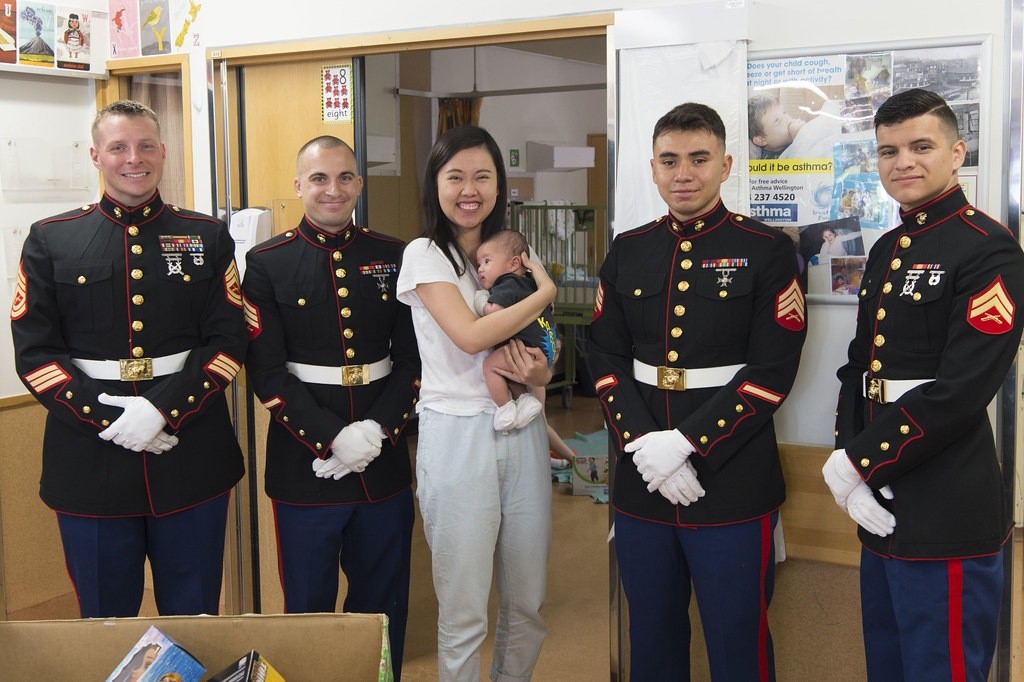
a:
[330,419,388,473]
[846,480,897,538]
[821,449,860,512]
[97,392,168,452]
[311,453,353,480]
[624,428,697,494]
[143,430,179,455]
[658,458,706,506]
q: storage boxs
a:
[1,611,396,682]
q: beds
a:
[509,198,600,413]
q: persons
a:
[585,104,809,681]
[241,135,421,681]
[536,331,577,463]
[394,125,565,681]
[745,41,981,310]
[818,89,1024,681]
[473,229,559,431]
[7,98,250,621]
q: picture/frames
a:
[748,31,994,303]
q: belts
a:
[69,350,190,382]
[286,354,393,386]
[864,369,936,406]
[633,357,747,391]
